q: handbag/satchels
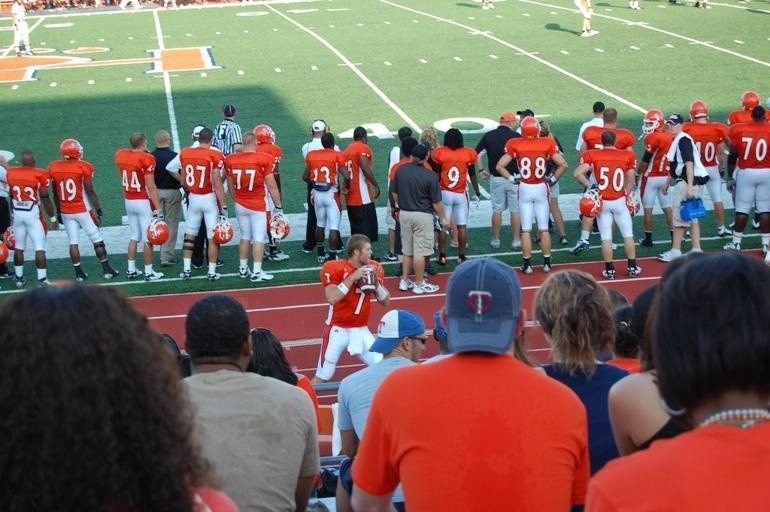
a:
[312,181,333,191]
[680,195,706,222]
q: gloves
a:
[591,184,599,189]
[545,173,558,187]
[96,209,103,226]
[719,165,725,178]
[635,173,640,186]
[157,209,165,221]
[221,206,229,218]
[472,194,481,209]
[508,173,521,185]
[56,210,63,225]
[726,178,736,194]
[273,206,284,214]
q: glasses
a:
[250,327,270,333]
[313,119,326,125]
[408,336,428,344]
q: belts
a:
[494,174,514,177]
[421,210,433,213]
[676,179,683,183]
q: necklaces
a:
[700,408,766,428]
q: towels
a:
[666,132,710,185]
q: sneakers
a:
[206,260,225,267]
[481,5,489,10]
[144,271,164,281]
[399,279,413,290]
[15,275,27,288]
[760,244,769,256]
[457,258,468,264]
[488,239,500,248]
[718,225,733,237]
[17,52,23,56]
[383,252,398,261]
[692,0,713,9]
[126,269,143,278]
[324,255,336,262]
[751,219,760,230]
[412,280,439,294]
[179,270,192,280]
[37,277,52,287]
[317,249,329,263]
[238,266,252,278]
[533,235,541,243]
[581,30,592,37]
[337,245,346,253]
[681,237,686,245]
[685,230,690,237]
[423,263,438,278]
[192,262,203,269]
[263,249,270,258]
[450,240,468,248]
[591,227,600,234]
[371,255,380,262]
[300,247,312,253]
[728,221,735,227]
[0,271,15,279]
[560,236,569,245]
[628,6,637,10]
[591,30,599,35]
[627,266,642,278]
[511,240,522,250]
[267,250,289,261]
[26,51,35,55]
[435,243,439,249]
[682,248,704,257]
[520,257,533,274]
[657,248,682,262]
[602,268,616,279]
[612,243,617,250]
[439,256,446,265]
[160,260,177,266]
[542,256,553,272]
[549,227,555,233]
[208,272,221,281]
[723,240,742,253]
[569,239,590,255]
[250,270,274,282]
[488,4,494,8]
[634,6,641,9]
[76,272,88,282]
[638,238,653,247]
[104,270,120,280]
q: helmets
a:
[689,100,709,122]
[147,219,169,245]
[60,139,83,159]
[642,109,665,134]
[580,188,602,218]
[0,240,8,264]
[269,213,290,240]
[3,226,15,250]
[253,125,275,144]
[212,221,233,244]
[521,116,540,138]
[626,198,640,216]
[741,91,759,110]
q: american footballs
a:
[357,266,377,293]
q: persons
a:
[224,131,284,283]
[658,114,709,262]
[49,139,119,281]
[421,128,441,173]
[212,105,243,194]
[575,102,614,237]
[534,122,568,245]
[681,100,733,236]
[529,270,634,477]
[496,117,569,274]
[607,304,648,373]
[249,328,322,491]
[305,133,351,263]
[475,111,522,251]
[165,126,227,268]
[342,126,381,262]
[386,125,426,262]
[251,125,290,262]
[637,108,685,247]
[0,153,8,268]
[592,287,631,377]
[308,233,384,383]
[179,128,227,281]
[391,146,449,292]
[587,255,770,511]
[7,151,58,289]
[339,308,430,511]
[570,108,636,253]
[114,133,163,281]
[606,284,693,456]
[583,130,642,279]
[302,119,343,255]
[727,92,770,231]
[0,283,237,512]
[388,138,423,263]
[149,131,182,266]
[352,256,593,510]
[723,107,770,255]
[514,110,538,249]
[432,129,480,265]
[174,293,322,511]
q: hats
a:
[500,111,519,123]
[434,310,448,340]
[517,109,535,116]
[369,307,425,355]
[222,104,235,115]
[192,125,205,137]
[445,257,521,354]
[313,121,325,132]
[411,142,430,159]
[666,114,683,125]
